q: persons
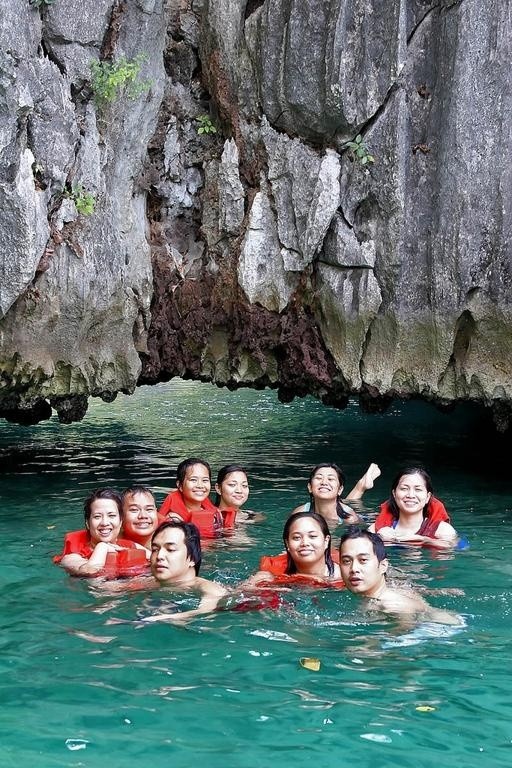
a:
[291,462,381,523]
[339,525,424,610]
[254,512,341,581]
[54,457,252,592]
[366,467,456,543]
